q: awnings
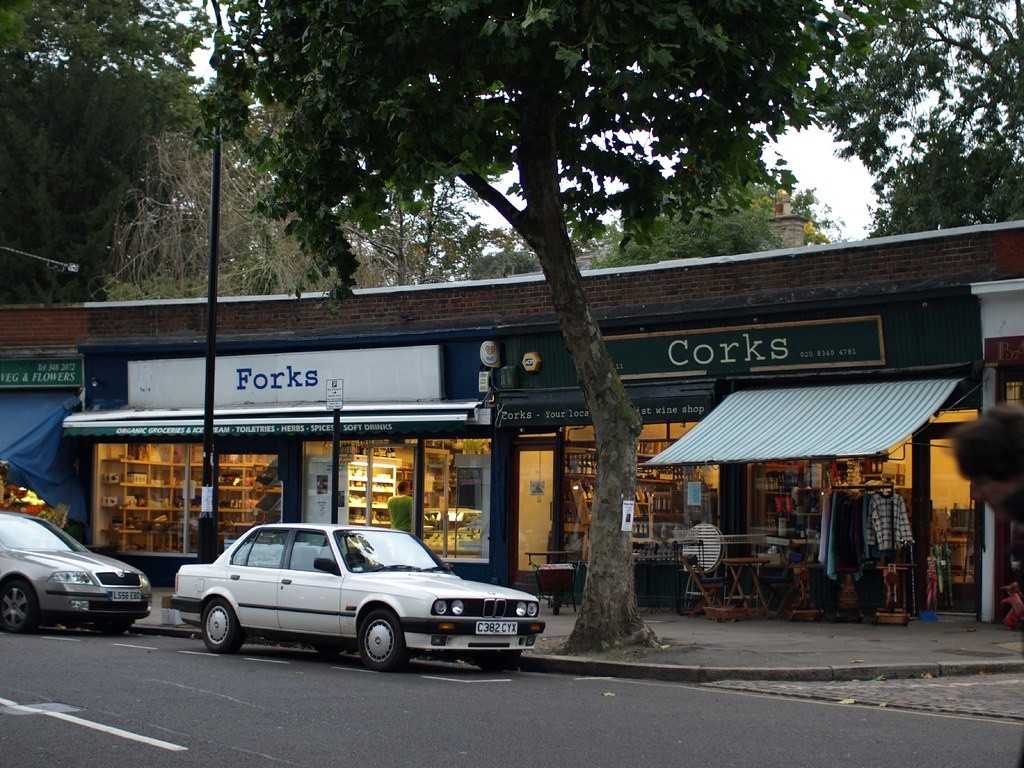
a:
[642,378,962,467]
[0,387,85,520]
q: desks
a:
[719,557,773,620]
[417,507,482,560]
[786,562,823,623]
[871,563,917,628]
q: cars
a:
[0,510,154,635]
[170,522,546,674]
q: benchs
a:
[224,539,311,570]
[762,582,793,621]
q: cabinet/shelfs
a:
[422,447,458,507]
[304,460,397,532]
[746,454,888,557]
[99,442,282,556]
[562,444,719,559]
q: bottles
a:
[800,525,805,539]
[776,510,787,539]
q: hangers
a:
[824,486,903,498]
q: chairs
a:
[682,553,726,622]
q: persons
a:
[387,481,412,533]
[952,403,1024,768]
